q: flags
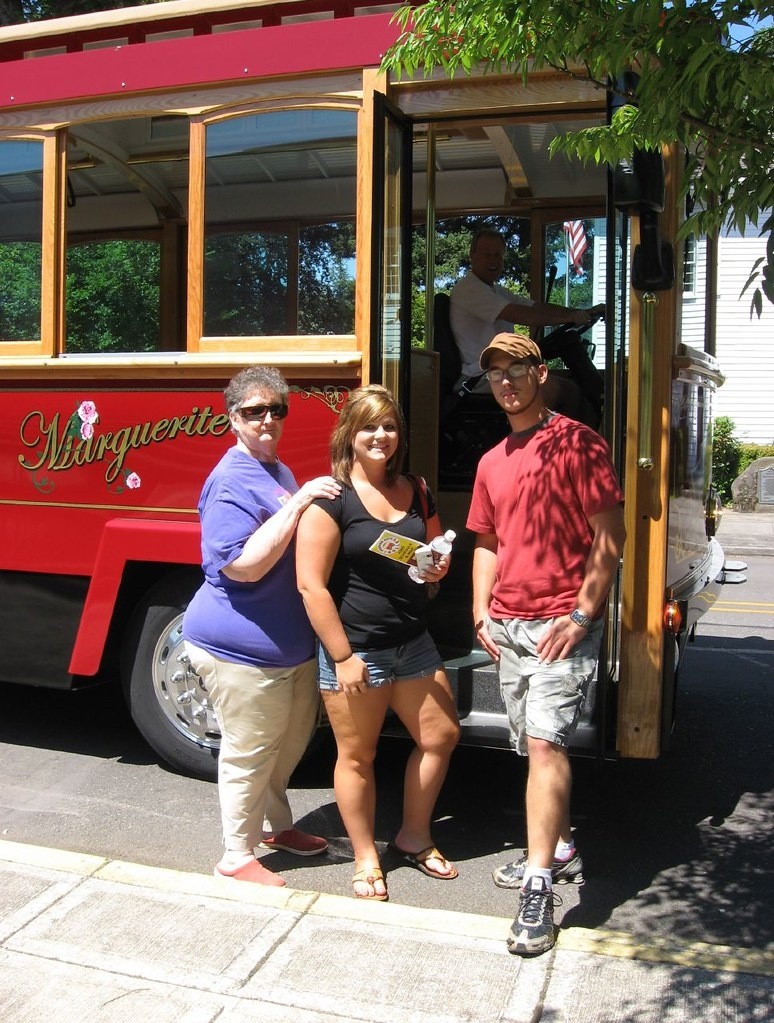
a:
[563,220,589,276]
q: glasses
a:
[235,403,288,422]
[484,361,537,383]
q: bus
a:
[0,0,727,783]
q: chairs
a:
[433,291,513,489]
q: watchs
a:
[570,609,591,629]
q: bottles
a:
[409,530,457,584]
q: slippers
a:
[352,867,389,901]
[387,838,458,879]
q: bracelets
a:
[333,652,352,663]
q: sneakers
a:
[507,875,563,954]
[494,846,585,888]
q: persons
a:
[465,332,624,956]
[293,384,460,900]
[182,363,327,889]
[447,228,593,420]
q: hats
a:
[479,333,541,372]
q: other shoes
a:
[213,857,285,888]
[258,825,329,856]
